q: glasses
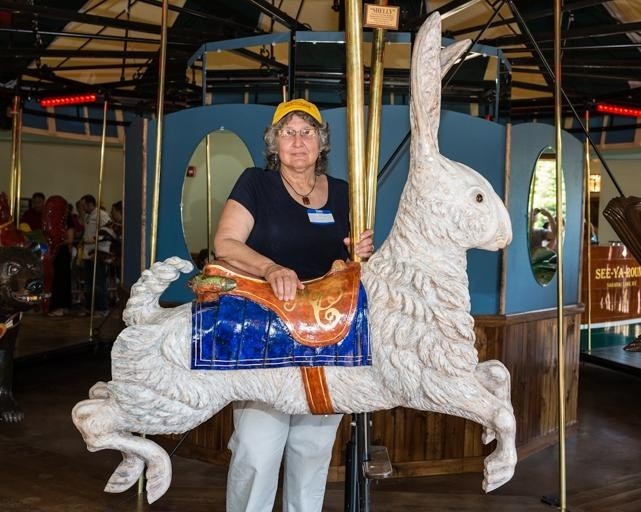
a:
[276,128,316,137]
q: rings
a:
[372,244,375,253]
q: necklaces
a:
[280,172,317,204]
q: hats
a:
[272,99,322,126]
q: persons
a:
[213,98,374,512]
[529,207,564,251]
[21,192,122,318]
[584,222,598,244]
